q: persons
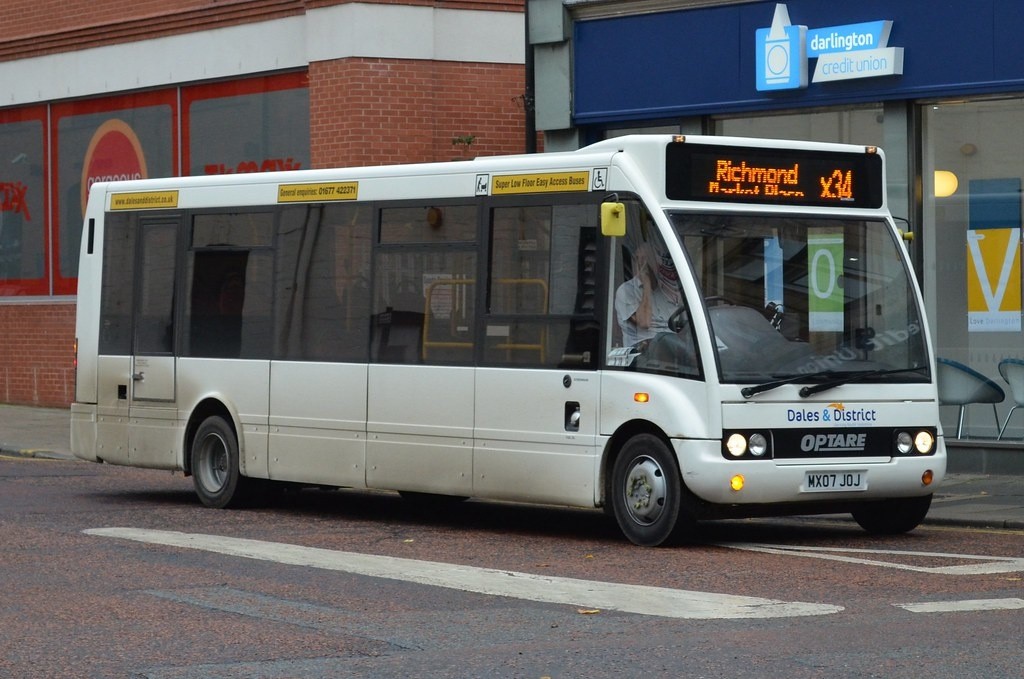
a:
[615,227,685,351]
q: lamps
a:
[934,170,959,198]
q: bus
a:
[72,131,949,550]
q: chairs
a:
[935,356,1005,440]
[997,358,1024,441]
